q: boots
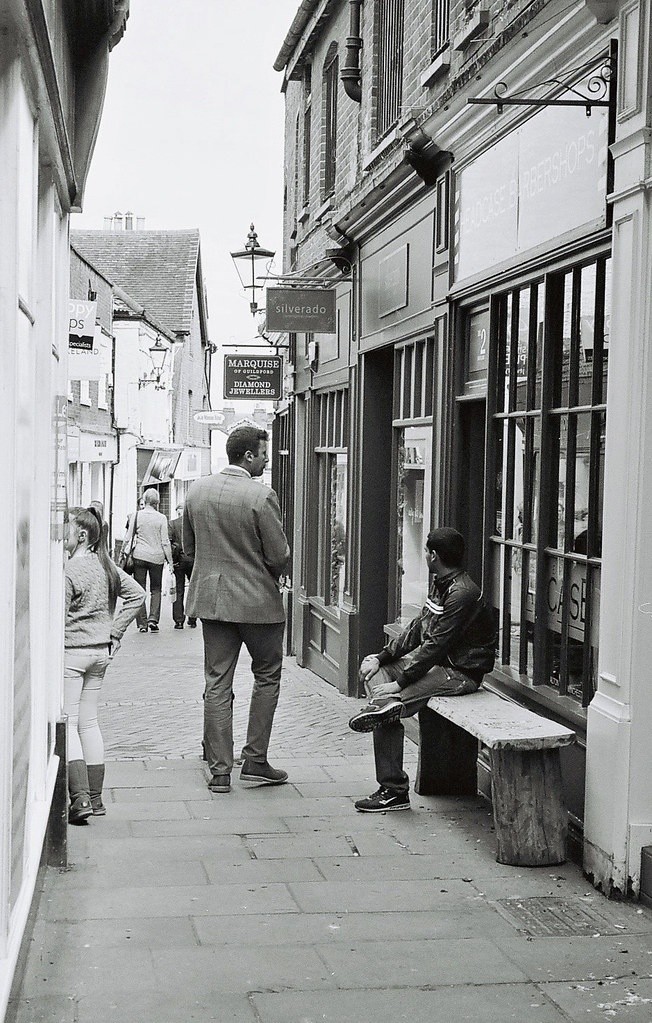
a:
[86,763,107,815]
[68,759,94,823]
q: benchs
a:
[415,687,576,866]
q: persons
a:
[556,503,566,550]
[125,497,146,550]
[63,506,149,823]
[117,487,175,633]
[168,501,198,629]
[91,501,108,555]
[181,425,290,793]
[347,526,498,813]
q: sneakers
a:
[349,698,406,733]
[355,785,411,811]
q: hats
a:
[427,527,464,562]
[175,503,184,511]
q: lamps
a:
[137,332,168,390]
[231,222,275,317]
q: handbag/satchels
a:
[123,547,135,575]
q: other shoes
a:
[140,628,148,632]
[149,621,159,630]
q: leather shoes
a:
[239,759,288,782]
[188,621,196,628]
[175,622,183,629]
[208,774,231,792]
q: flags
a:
[69,324,102,382]
[69,299,98,351]
